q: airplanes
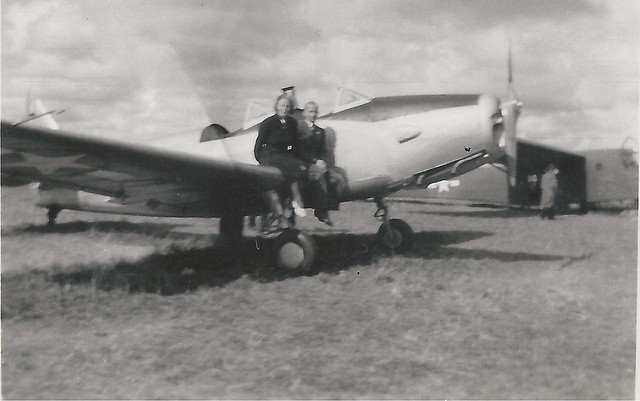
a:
[0,38,524,274]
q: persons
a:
[254,96,308,219]
[539,164,559,220]
[296,102,346,227]
